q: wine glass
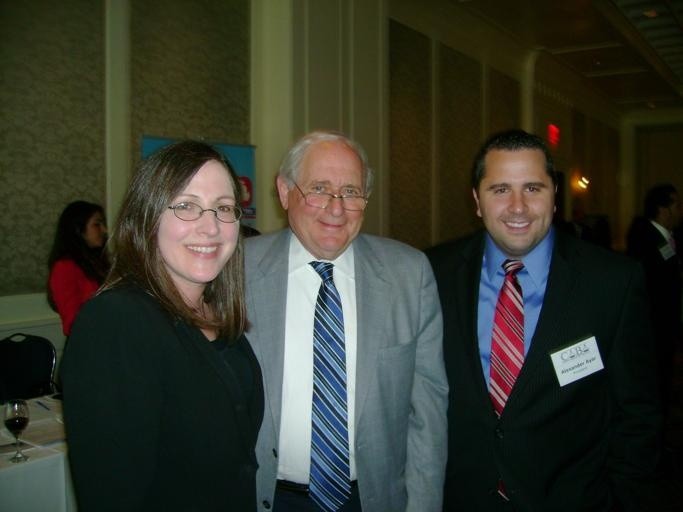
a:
[3,399,30,462]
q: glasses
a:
[163,199,245,223]
[289,176,372,212]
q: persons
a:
[422,124,677,510]
[625,180,681,280]
[45,199,112,337]
[240,130,450,510]
[55,140,266,511]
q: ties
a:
[488,258,527,417]
[306,258,351,512]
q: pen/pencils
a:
[36,401,51,411]
